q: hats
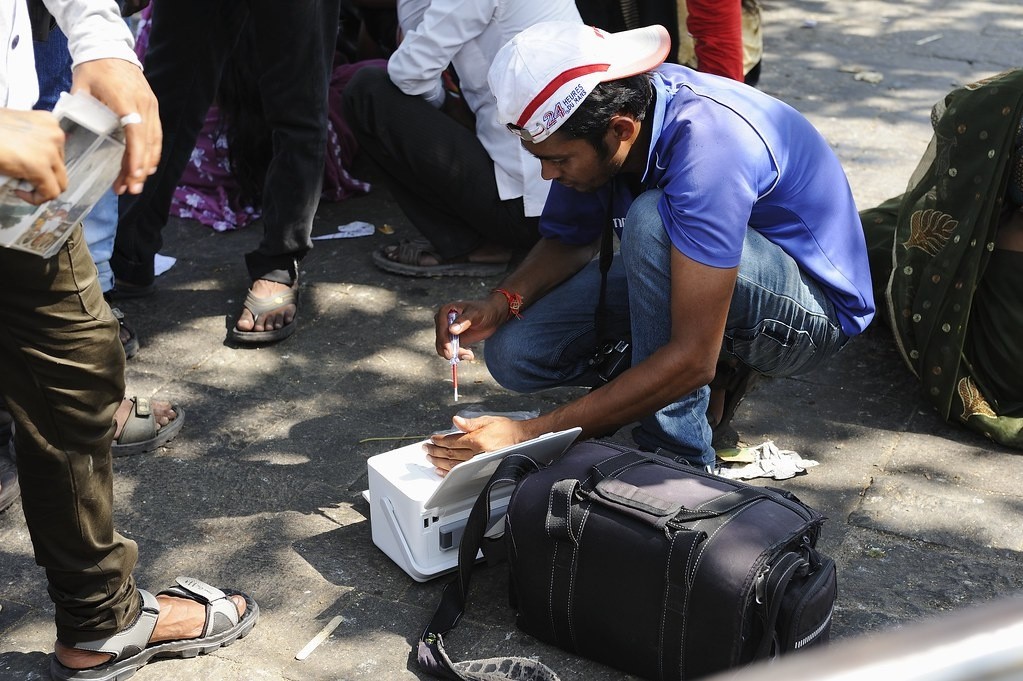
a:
[486,19,671,146]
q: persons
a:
[0,399,186,463]
[24,0,151,363]
[1,138,115,256]
[425,21,877,477]
[857,68,1022,454]
[578,0,765,91]
[100,0,346,344]
[341,0,596,279]
[0,0,258,681]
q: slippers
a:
[370,236,510,278]
[103,280,156,305]
[231,284,299,342]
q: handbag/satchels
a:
[410,434,840,681]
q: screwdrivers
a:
[448,309,462,403]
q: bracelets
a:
[488,287,524,321]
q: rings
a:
[120,112,141,128]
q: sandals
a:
[10,397,186,464]
[703,356,761,445]
[102,293,139,360]
[52,575,262,681]
